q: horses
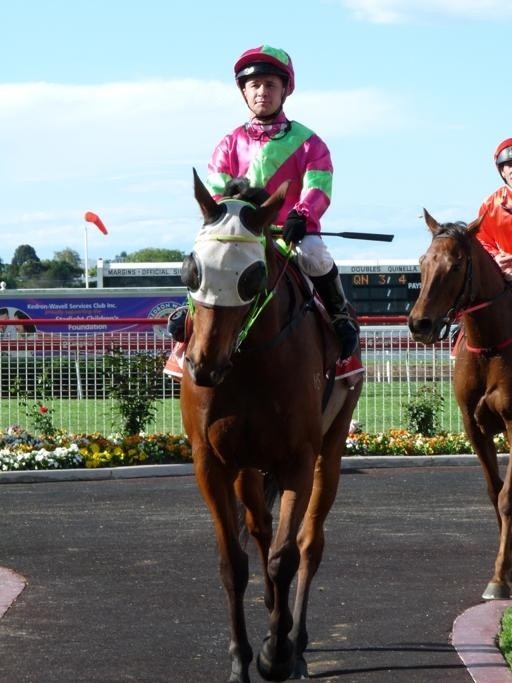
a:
[179,165,366,683]
[405,206,511,604]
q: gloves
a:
[239,187,270,204]
[282,213,306,245]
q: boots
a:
[309,261,359,360]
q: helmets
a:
[494,139,512,171]
[233,46,294,96]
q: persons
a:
[1,307,12,334]
[14,309,36,338]
[464,133,512,275]
[158,42,363,363]
